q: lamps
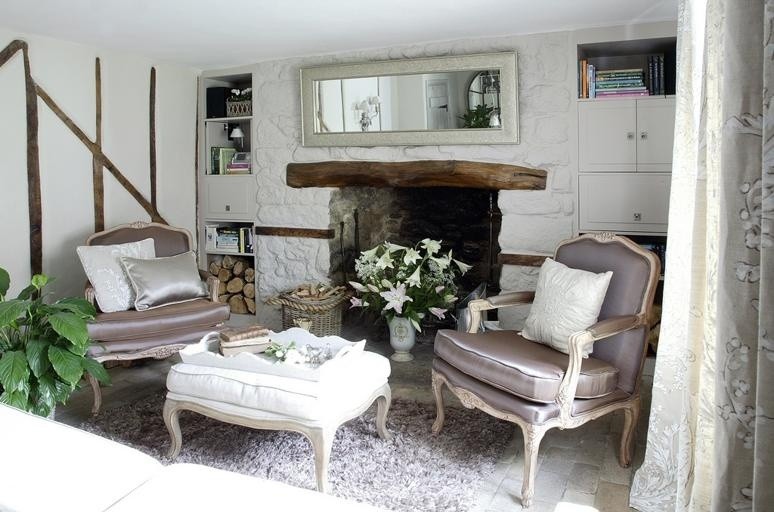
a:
[230,126,245,148]
[358,96,382,132]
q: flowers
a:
[349,238,473,336]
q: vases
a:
[384,315,416,363]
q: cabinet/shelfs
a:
[573,21,677,378]
[196,64,260,328]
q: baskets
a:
[264,281,355,337]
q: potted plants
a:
[0,268,114,421]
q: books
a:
[219,326,272,357]
[639,244,666,274]
[207,224,253,254]
[213,147,251,174]
[579,54,667,97]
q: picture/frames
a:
[211,147,223,175]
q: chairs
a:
[82,221,231,417]
[431,232,662,509]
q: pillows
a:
[118,250,211,313]
[516,257,614,359]
[76,237,156,312]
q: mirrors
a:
[298,50,520,147]
[462,70,501,121]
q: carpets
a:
[78,387,518,512]
